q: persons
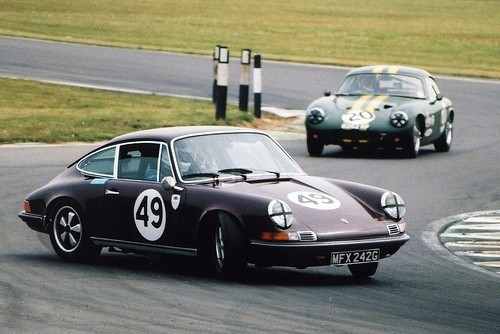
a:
[145,146,203,181]
[354,76,380,94]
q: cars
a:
[304,65,456,159]
[18,125,410,284]
